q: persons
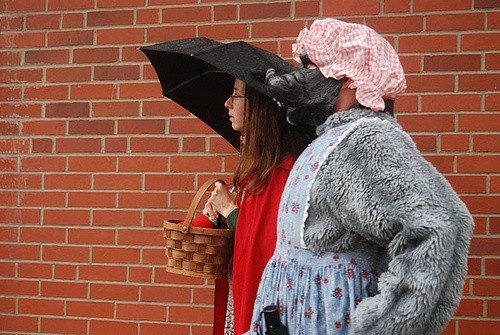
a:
[240,16,476,335]
[162,76,303,335]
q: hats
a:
[292,18,407,112]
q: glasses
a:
[229,95,249,103]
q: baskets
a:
[163,177,234,279]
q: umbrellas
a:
[134,37,301,156]
